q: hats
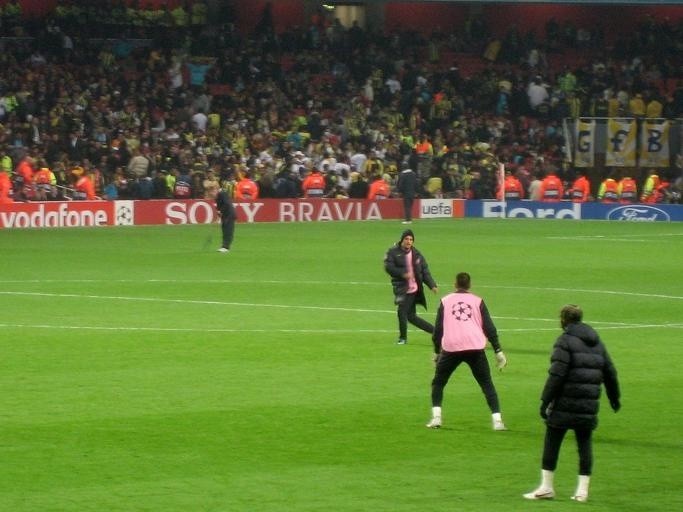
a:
[402,228,415,242]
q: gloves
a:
[540,402,549,420]
[494,350,508,371]
[610,401,622,413]
[431,352,441,368]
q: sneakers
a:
[571,495,587,503]
[493,426,509,430]
[523,491,554,500]
[215,247,230,252]
[397,339,406,345]
[426,421,441,428]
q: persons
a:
[208,186,237,252]
[384,228,439,344]
[521,303,621,501]
[426,272,507,430]
[396,164,417,224]
